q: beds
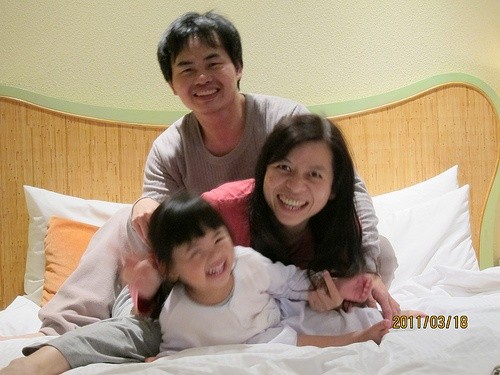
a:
[0,70,500,375]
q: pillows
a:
[22,184,133,298]
[372,164,458,217]
[377,184,480,283]
[41,216,99,308]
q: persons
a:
[0,10,401,346]
[0,115,365,374]
[143,193,430,363]
